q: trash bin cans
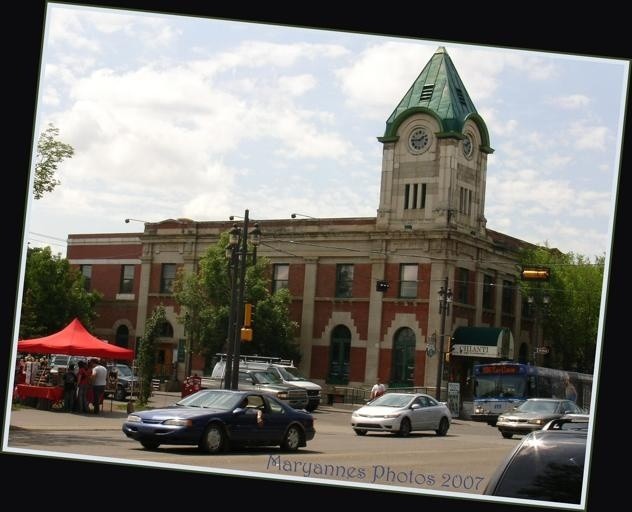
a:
[327,394,334,405]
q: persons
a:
[562,374,578,403]
[60,364,78,413]
[90,359,108,414]
[15,353,49,405]
[84,358,108,384]
[370,378,385,401]
[75,361,91,414]
[239,397,264,430]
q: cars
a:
[349,388,455,440]
[121,385,317,455]
[482,395,592,504]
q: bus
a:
[466,361,594,424]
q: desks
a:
[16,383,105,410]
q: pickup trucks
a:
[187,368,309,414]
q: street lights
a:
[433,276,455,404]
[218,222,263,391]
[527,287,550,364]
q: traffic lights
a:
[449,337,455,350]
[448,353,452,362]
[376,281,390,293]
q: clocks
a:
[406,124,432,155]
[462,126,477,160]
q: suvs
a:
[46,355,151,402]
[211,350,322,411]
